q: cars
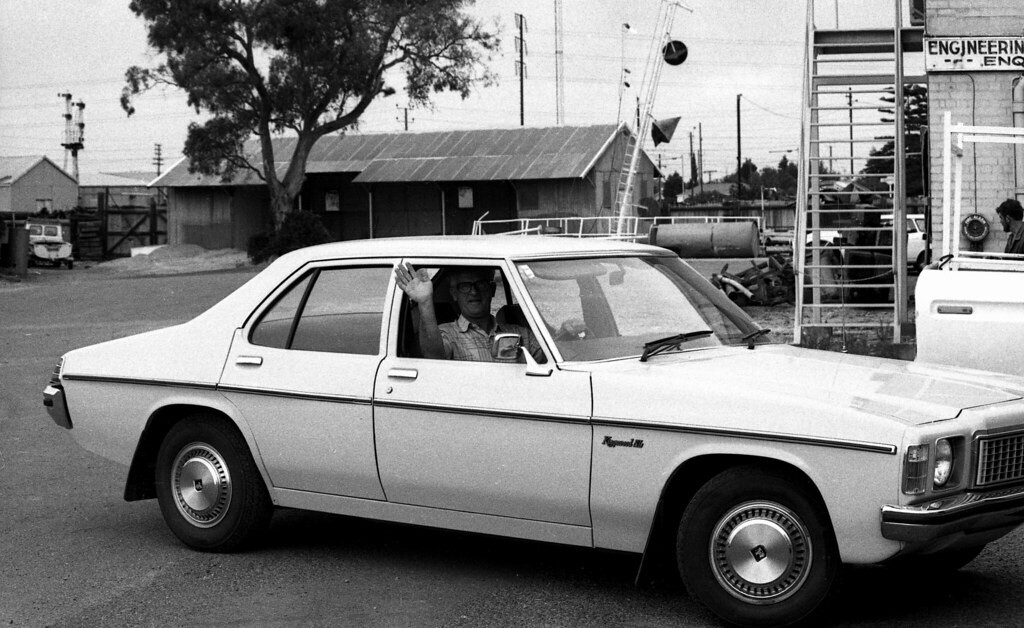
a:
[39,236,1024,628]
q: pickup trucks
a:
[912,254,1024,377]
[805,214,933,274]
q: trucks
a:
[26,223,74,270]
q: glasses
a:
[457,280,489,294]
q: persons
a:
[395,262,587,365]
[987,198,1024,261]
